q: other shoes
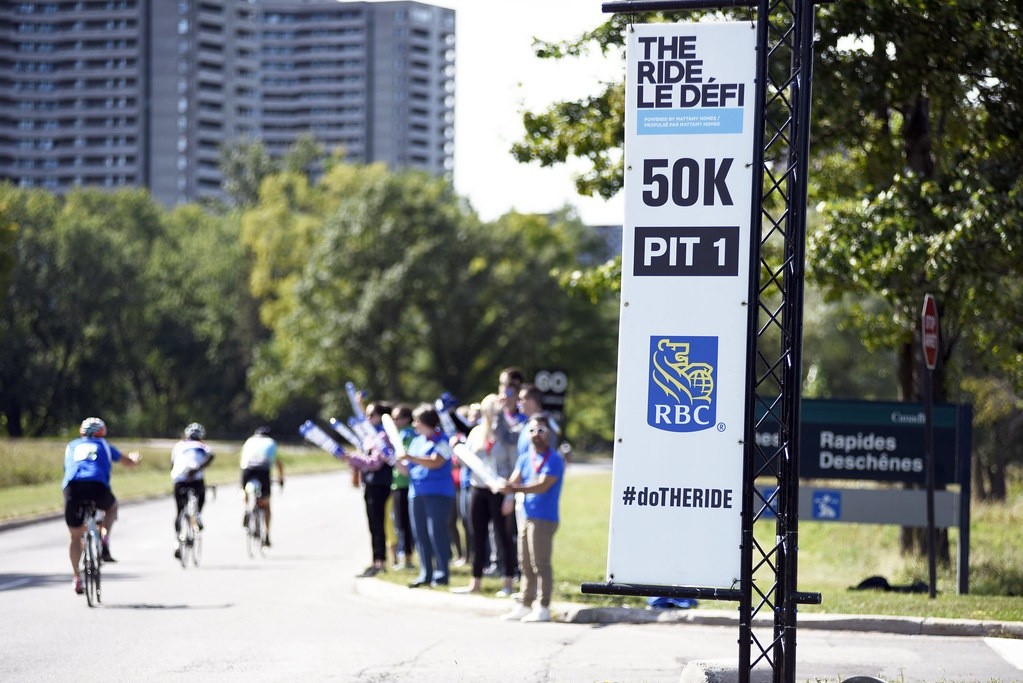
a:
[74,578,84,594]
[263,534,270,545]
[101,536,111,562]
[193,516,204,530]
[174,548,181,559]
[243,512,251,527]
[358,549,551,622]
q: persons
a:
[240,427,284,545]
[62,417,142,593]
[341,367,564,622]
[171,423,214,557]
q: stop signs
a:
[922,293,939,371]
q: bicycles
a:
[242,477,284,558]
[70,498,119,608]
[178,484,217,569]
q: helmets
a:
[79,417,106,436]
[254,426,270,436]
[185,423,206,441]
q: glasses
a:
[529,427,549,433]
